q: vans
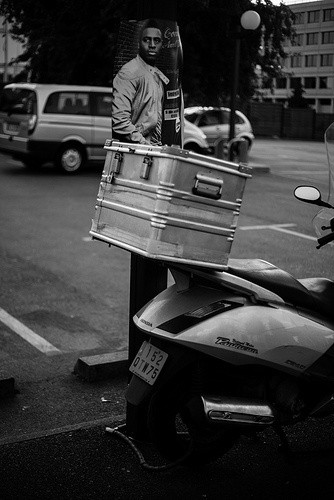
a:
[0,84,210,175]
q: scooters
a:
[106,122,334,479]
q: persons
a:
[112,24,184,149]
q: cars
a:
[184,106,254,156]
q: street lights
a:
[228,10,261,142]
